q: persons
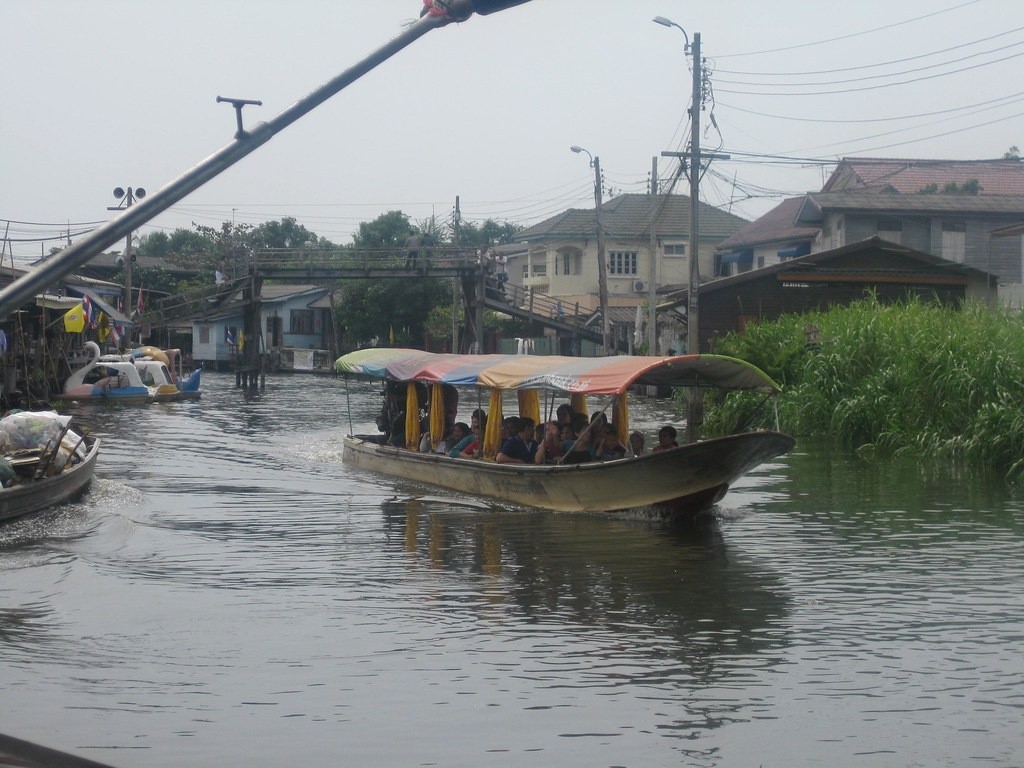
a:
[478,243,507,292]
[404,231,419,269]
[214,255,227,284]
[553,300,564,321]
[380,380,457,446]
[420,233,436,268]
[420,404,678,466]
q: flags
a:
[240,330,245,349]
[63,288,143,347]
[226,329,234,345]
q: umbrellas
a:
[633,306,643,354]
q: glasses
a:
[558,413,570,417]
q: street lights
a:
[571,145,610,357]
[653,15,701,440]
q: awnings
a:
[778,243,811,257]
[69,285,134,327]
[721,249,753,262]
[36,295,81,309]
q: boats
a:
[62,342,204,406]
[264,347,337,373]
[337,347,797,521]
[0,407,102,521]
